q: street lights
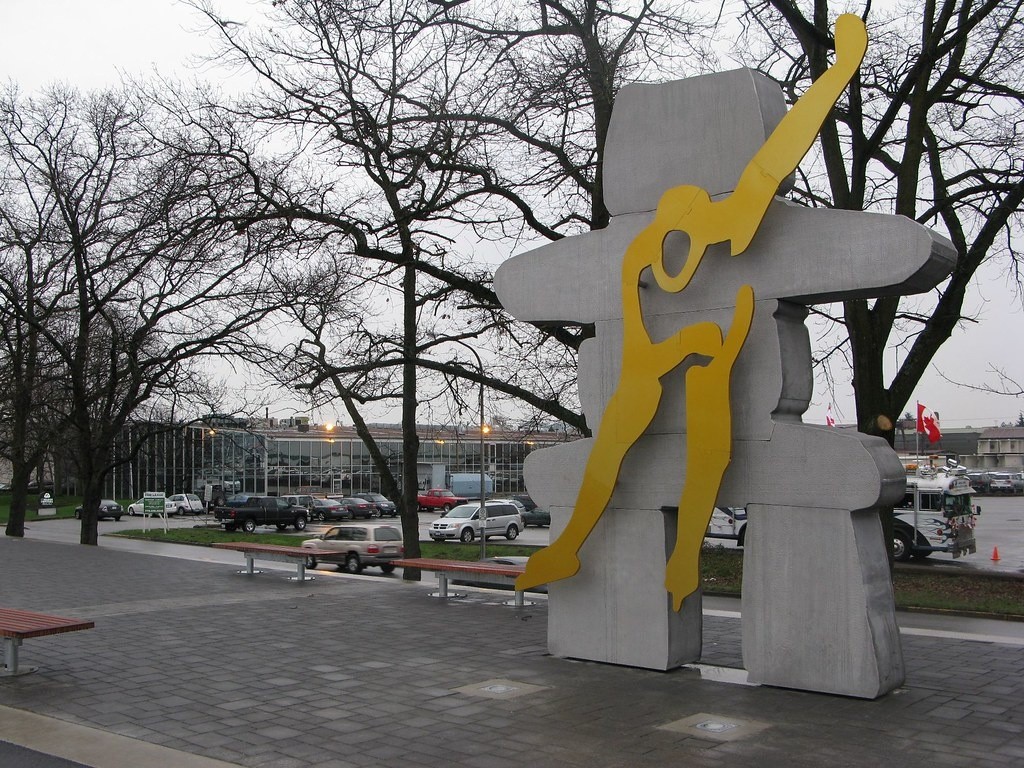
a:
[426,331,485,562]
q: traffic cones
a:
[989,546,1000,561]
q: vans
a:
[281,494,318,518]
[196,466,241,492]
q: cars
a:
[0,480,55,495]
[246,466,368,485]
[74,499,123,522]
[308,497,376,521]
[966,470,1024,494]
[490,472,520,485]
[485,494,551,527]
[169,493,204,516]
[127,494,176,518]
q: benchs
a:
[212,542,347,581]
[389,558,536,606]
[0,609,94,676]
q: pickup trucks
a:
[416,489,470,512]
[214,496,310,534]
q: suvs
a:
[349,491,397,518]
[301,523,403,573]
[428,502,524,543]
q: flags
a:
[917,404,940,444]
[826,404,835,427]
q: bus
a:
[704,459,980,562]
